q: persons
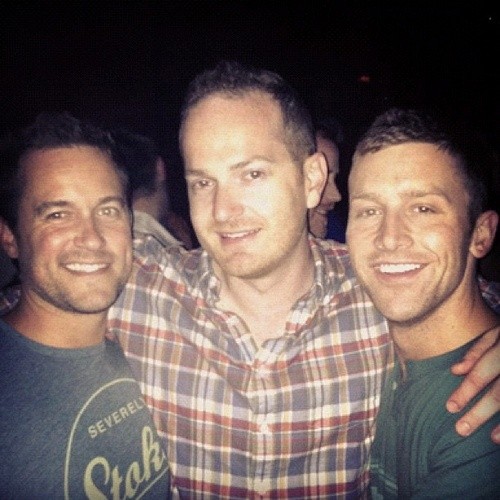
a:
[0,60,500,499]
